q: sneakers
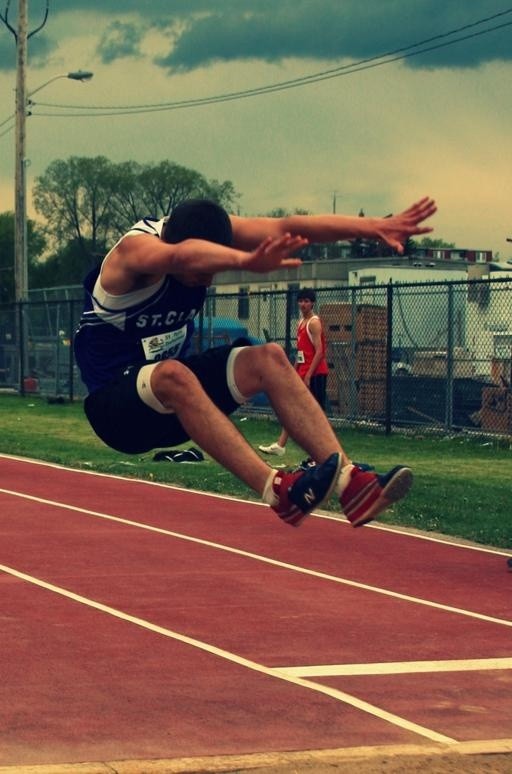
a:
[258,442,286,456]
[271,453,342,527]
[339,465,413,528]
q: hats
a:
[165,199,233,247]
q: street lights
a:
[12,70,95,390]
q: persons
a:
[258,288,330,456]
[73,196,437,527]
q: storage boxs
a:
[413,352,472,377]
[482,387,512,434]
[320,304,387,415]
